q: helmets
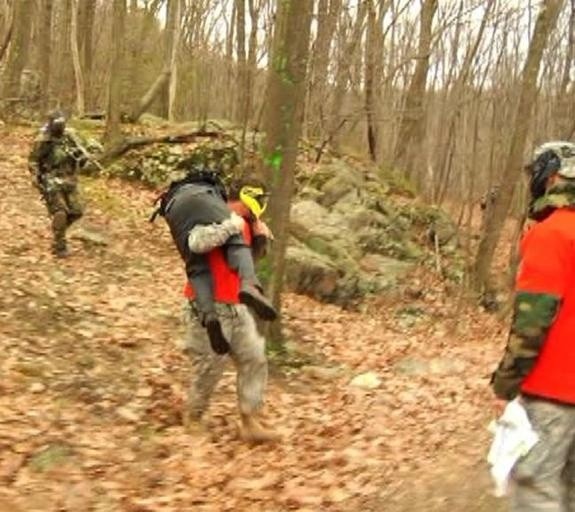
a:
[524,140,575,198]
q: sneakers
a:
[181,414,202,437]
[206,312,230,355]
[237,283,277,322]
[241,423,282,444]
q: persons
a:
[485,141,575,512]
[25,112,98,260]
[479,184,501,231]
[180,181,285,446]
[159,170,277,354]
